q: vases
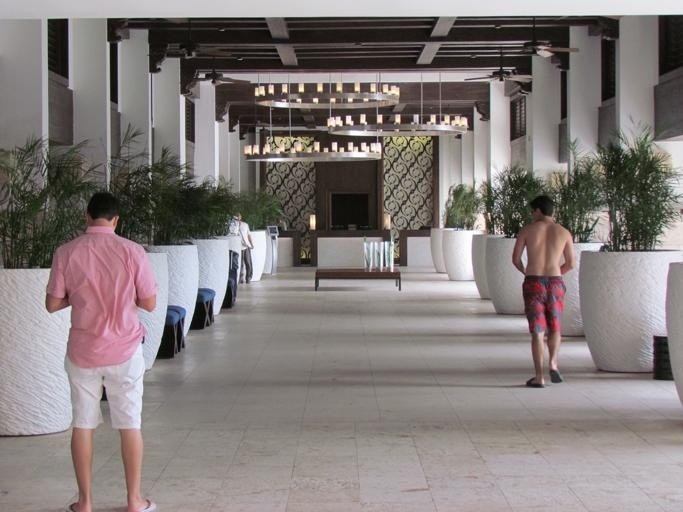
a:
[362,241,372,271]
[384,240,394,271]
[374,241,383,272]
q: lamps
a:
[241,72,468,162]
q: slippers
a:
[525,376,546,388]
[63,500,80,512]
[137,497,158,512]
[548,368,563,384]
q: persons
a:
[513,196,576,387]
[233,210,256,284]
[45,191,157,512]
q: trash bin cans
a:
[653,336,674,380]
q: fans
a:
[191,56,250,86]
[464,47,533,83]
[520,17,579,71]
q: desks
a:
[314,268,401,292]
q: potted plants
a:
[431,115,683,401]
[1,125,279,436]
